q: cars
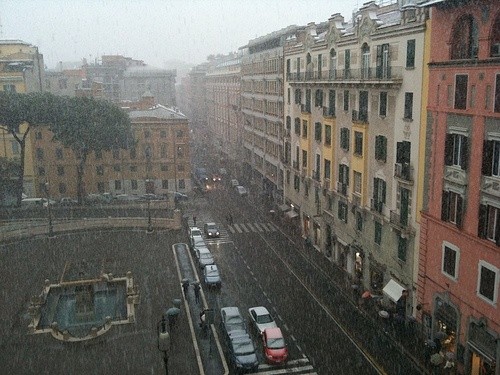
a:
[195,248,216,270]
[204,264,222,288]
[188,227,203,243]
[196,167,229,194]
[19,191,189,204]
[204,223,220,239]
[220,307,260,375]
[232,180,239,188]
[262,327,288,363]
[248,306,277,337]
[235,186,247,197]
[193,236,206,253]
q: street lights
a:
[45,183,51,229]
[156,315,171,375]
[145,179,152,232]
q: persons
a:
[229,217,232,225]
[425,339,449,375]
[194,284,200,304]
[193,217,197,226]
[444,358,457,375]
[182,281,189,298]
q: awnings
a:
[287,211,298,217]
[280,204,290,211]
[383,279,406,304]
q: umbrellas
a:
[424,339,436,346]
[379,310,389,319]
[431,353,443,365]
[446,352,456,358]
[362,289,371,298]
[435,332,450,340]
[351,285,360,290]
